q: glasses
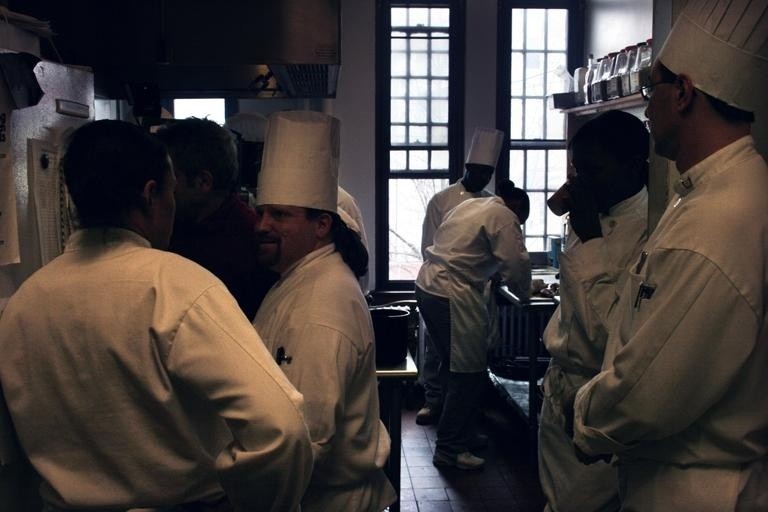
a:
[637,77,674,101]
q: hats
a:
[656,1,768,113]
[254,108,342,213]
[464,126,505,170]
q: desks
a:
[375,349,420,511]
[481,259,569,470]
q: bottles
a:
[630,42,647,94]
[640,39,653,68]
[601,46,636,100]
[583,54,595,105]
[591,58,602,103]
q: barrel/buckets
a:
[370,307,410,369]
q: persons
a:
[249,109,395,512]
[156,115,237,323]
[415,188,551,465]
[572,0,766,511]
[1,119,312,511]
[338,184,370,295]
[538,109,648,511]
[417,127,505,425]
